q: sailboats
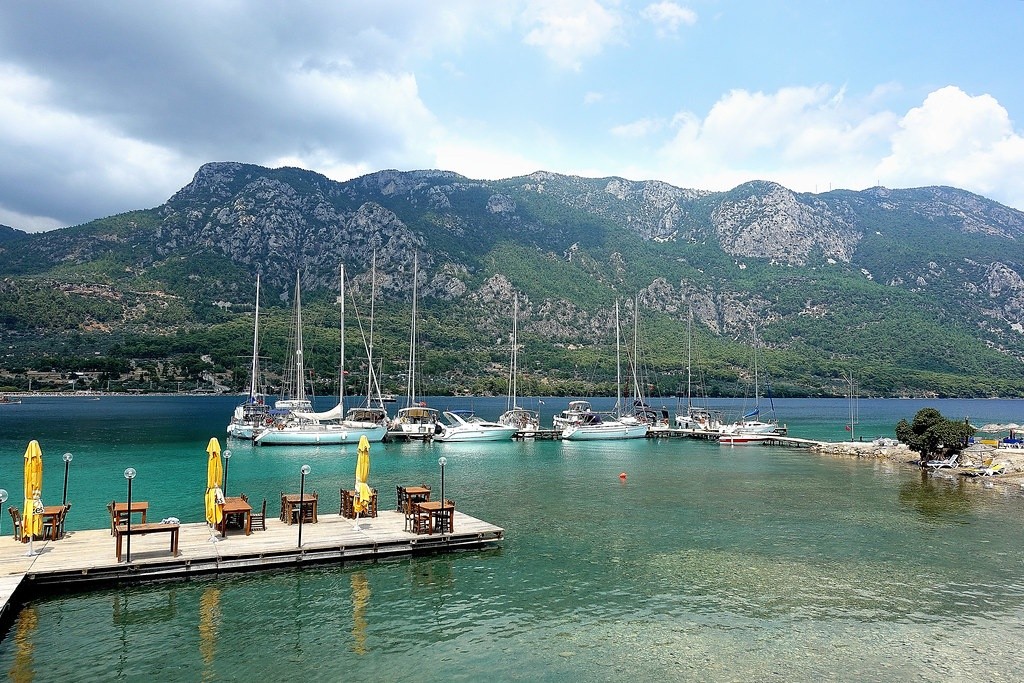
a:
[675,304,721,429]
[561,298,649,441]
[253,263,389,444]
[621,294,671,429]
[499,293,540,438]
[268,268,319,428]
[383,248,440,438]
[227,273,278,439]
[714,323,786,436]
[342,249,392,429]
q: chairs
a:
[207,492,266,531]
[8,504,71,543]
[280,490,318,526]
[396,485,455,535]
[111,501,128,537]
[339,488,378,520]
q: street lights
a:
[438,456,448,534]
[298,465,311,548]
[61,453,72,537]
[222,449,232,497]
[124,467,136,562]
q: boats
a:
[552,402,597,431]
[432,407,518,441]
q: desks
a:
[221,497,252,537]
[40,507,65,541]
[414,502,455,535]
[287,494,317,524]
[347,492,375,518]
[403,487,430,514]
[116,522,180,562]
[114,502,148,536]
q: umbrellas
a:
[23,441,44,551]
[963,422,1024,439]
[353,436,372,526]
[206,438,225,536]
